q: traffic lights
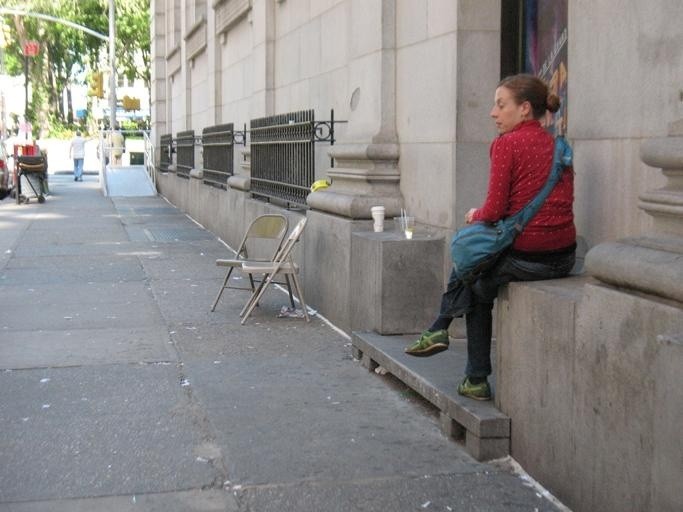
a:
[88,72,103,98]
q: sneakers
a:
[403,329,450,357]
[457,376,491,401]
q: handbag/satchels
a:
[450,217,512,281]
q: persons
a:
[400,72,578,403]
[67,129,95,182]
[108,124,125,167]
[95,131,109,166]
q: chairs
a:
[239,218,310,325]
[212,215,294,313]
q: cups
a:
[393,217,415,240]
[370,206,386,233]
